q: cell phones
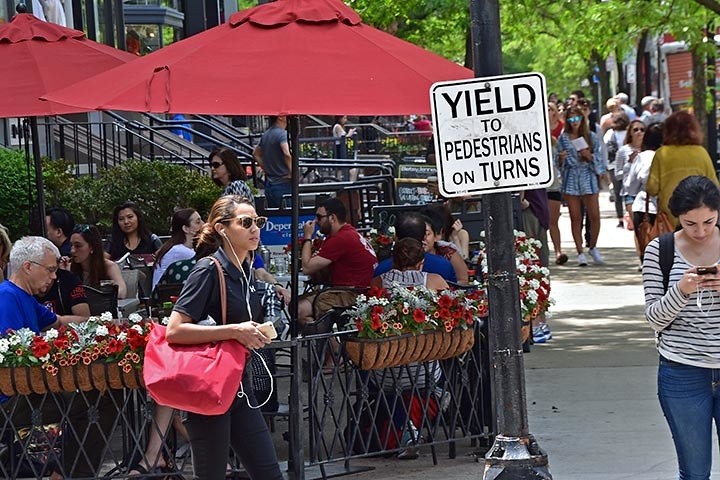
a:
[696,267,717,276]
[255,321,277,341]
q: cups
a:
[100,279,115,287]
[275,255,285,276]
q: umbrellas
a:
[0,11,142,234]
[37,0,475,463]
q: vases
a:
[345,328,474,364]
[0,356,149,398]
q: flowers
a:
[472,217,558,325]
[0,309,174,374]
[364,226,395,250]
[344,276,486,337]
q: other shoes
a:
[110,360,422,480]
[555,195,644,271]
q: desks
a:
[274,264,313,341]
[116,296,139,316]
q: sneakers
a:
[539,323,552,340]
[532,327,546,343]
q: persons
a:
[0,87,720,479]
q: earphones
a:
[220,229,227,238]
[697,296,702,309]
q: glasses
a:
[29,261,59,273]
[221,215,268,230]
[315,213,331,221]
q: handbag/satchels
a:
[638,192,675,266]
[143,255,250,416]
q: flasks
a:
[261,249,270,273]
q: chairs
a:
[120,269,139,298]
[300,285,369,377]
[78,280,119,318]
